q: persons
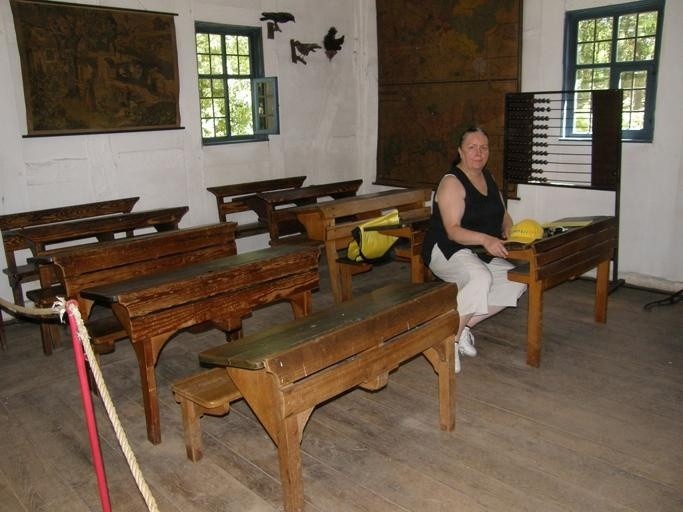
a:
[417,121,528,373]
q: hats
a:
[503,218,542,244]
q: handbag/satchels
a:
[348,210,400,263]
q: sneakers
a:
[455,328,477,373]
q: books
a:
[543,218,593,227]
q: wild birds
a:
[293,40,322,60]
[260,12,296,30]
